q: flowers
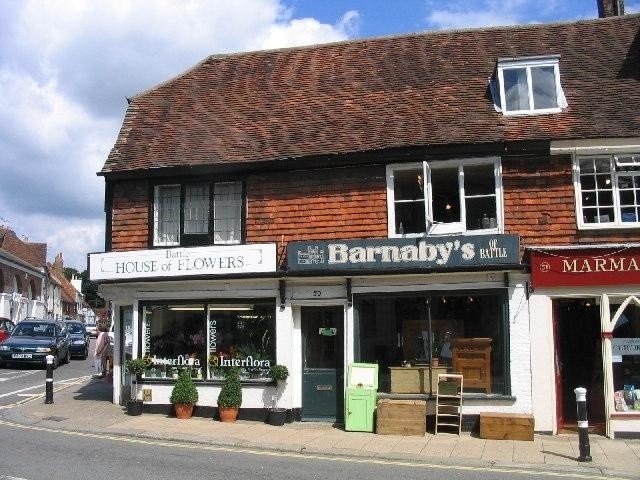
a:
[150,329,276,368]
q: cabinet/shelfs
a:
[450,338,493,392]
[346,387,377,433]
[435,374,463,435]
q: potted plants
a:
[267,365,290,426]
[169,369,242,422]
[123,356,153,415]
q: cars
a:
[0,316,98,370]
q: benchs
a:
[479,412,535,441]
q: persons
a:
[92,323,111,378]
